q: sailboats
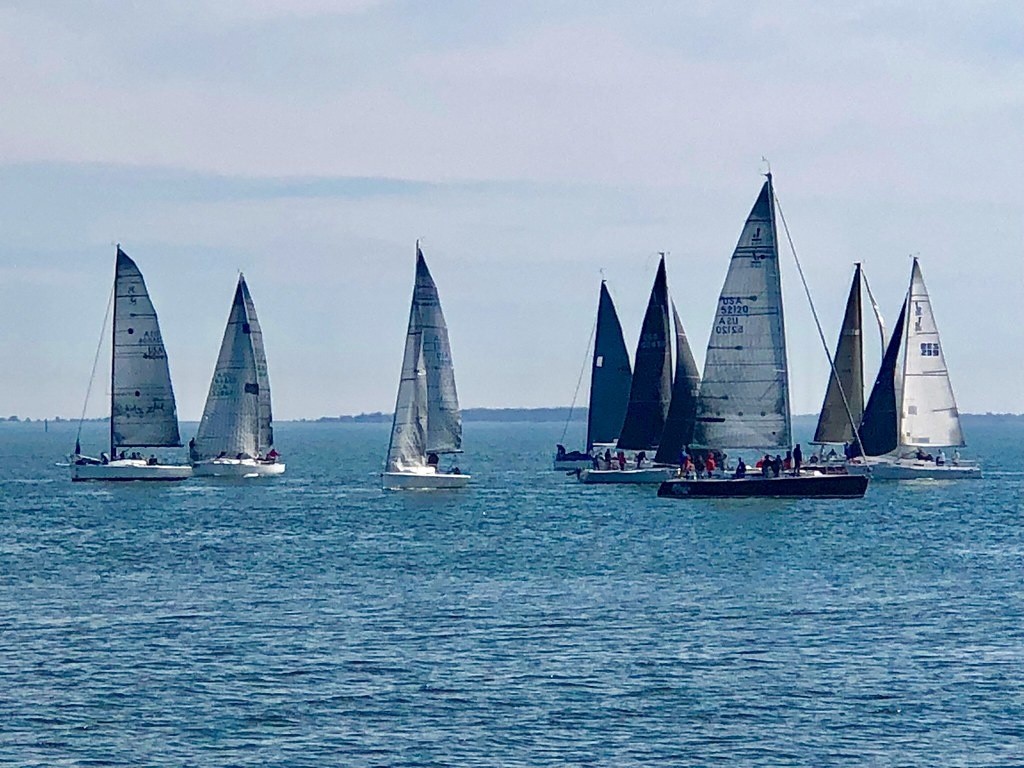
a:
[65,241,196,482]
[186,268,288,476]
[552,169,986,496]
[380,239,472,489]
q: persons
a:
[720,449,793,479]
[953,449,960,467]
[809,451,818,465]
[793,443,803,478]
[918,448,935,462]
[678,445,715,481]
[428,453,438,470]
[189,437,196,451]
[935,448,946,466]
[592,448,649,470]
[99,449,157,466]
[556,443,566,457]
[267,450,281,463]
